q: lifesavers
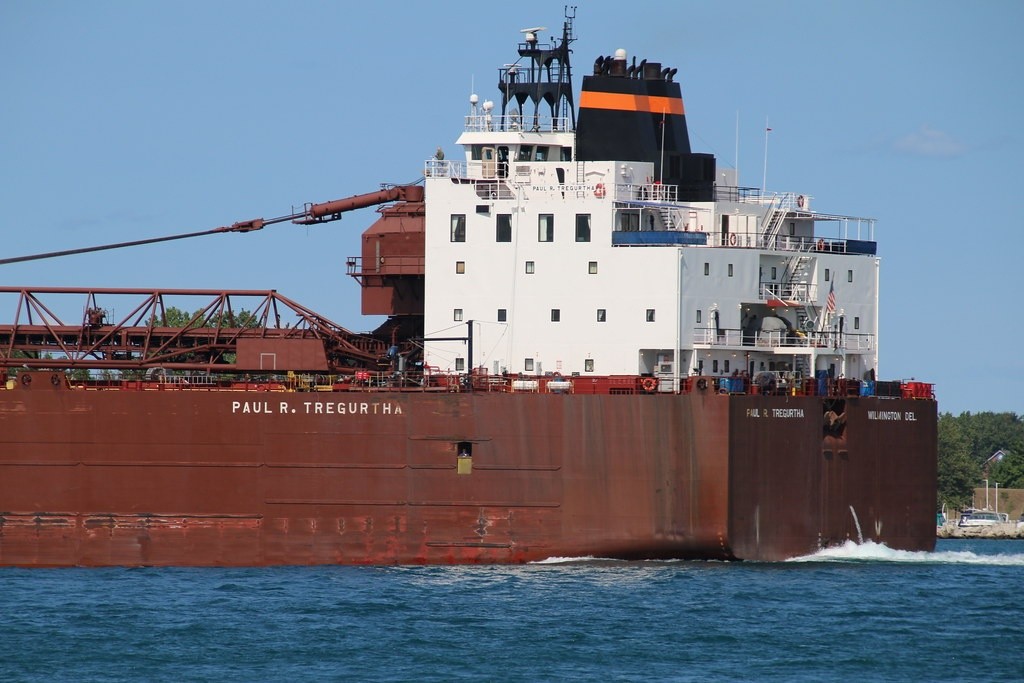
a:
[593,184,605,197]
[797,196,804,207]
[817,239,825,250]
[730,233,736,245]
[642,377,656,390]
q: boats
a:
[0,0,940,568]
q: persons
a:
[435,147,445,176]
